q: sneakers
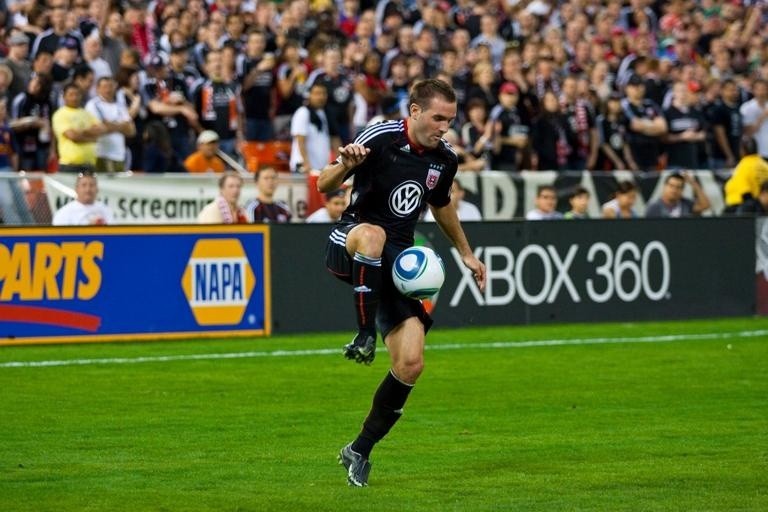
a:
[344,330,377,364]
[336,439,372,487]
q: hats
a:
[199,131,219,145]
[500,83,518,95]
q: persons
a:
[1,0,768,225]
[316,79,488,487]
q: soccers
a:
[391,246,446,298]
